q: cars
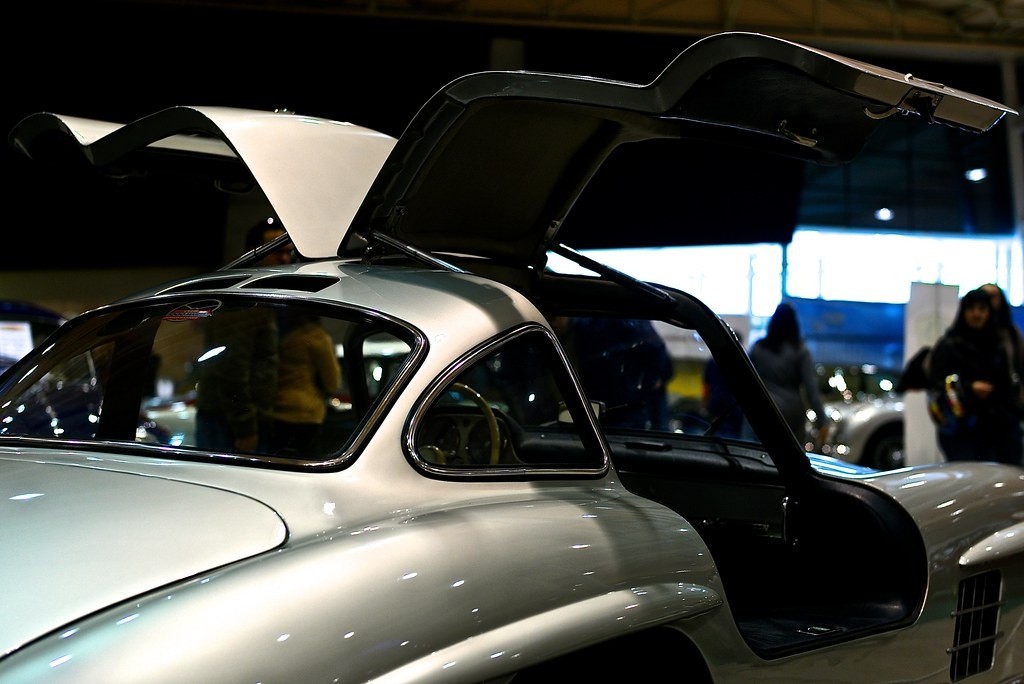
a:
[0,30,1024,684]
[814,394,907,471]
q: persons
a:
[543,314,675,435]
[748,302,829,454]
[925,283,1024,475]
[188,217,292,458]
[703,330,750,442]
[248,299,340,463]
